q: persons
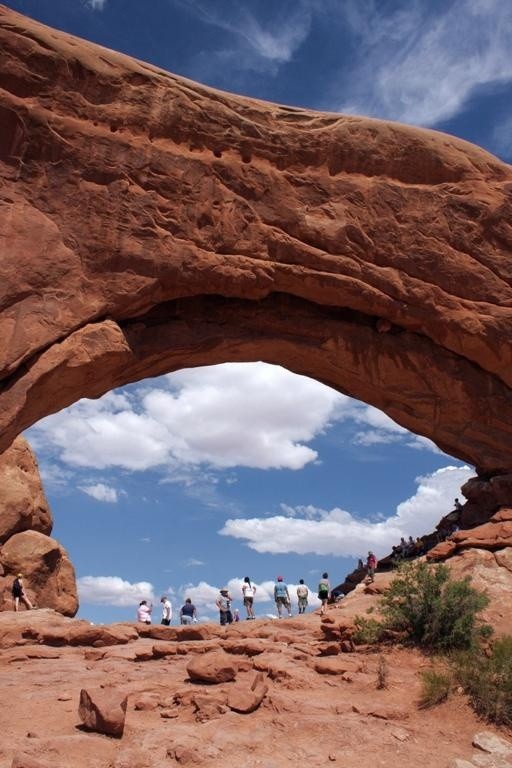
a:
[160,596,173,626]
[367,550,377,580]
[389,497,465,567]
[331,589,344,602]
[136,600,152,624]
[180,598,196,626]
[273,575,292,618]
[317,572,330,616]
[241,576,257,620]
[12,574,34,612]
[233,609,239,622]
[296,579,308,614]
[216,589,234,625]
